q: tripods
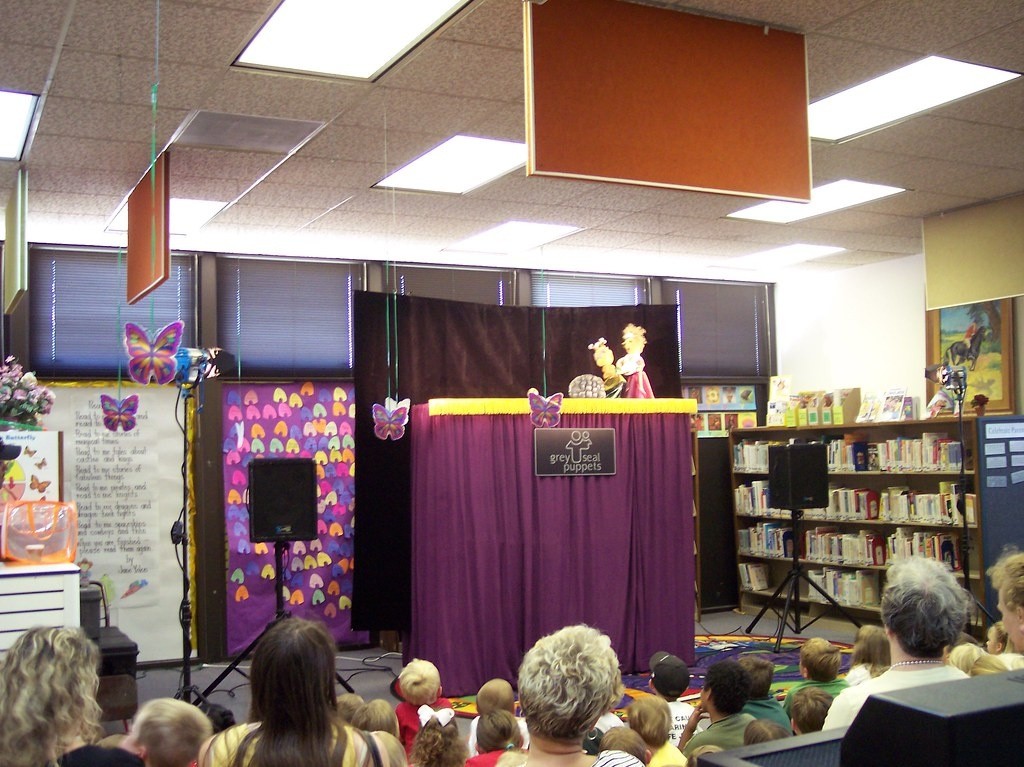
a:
[746,508,863,653]
[191,541,355,706]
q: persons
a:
[337,658,530,767]
[684,716,792,767]
[647,651,705,751]
[943,552,1024,677]
[0,626,145,767]
[782,637,850,721]
[464,624,625,767]
[677,660,758,752]
[628,694,690,767]
[197,617,409,767]
[822,555,972,730]
[843,625,892,686]
[790,686,834,736]
[96,696,238,767]
[738,656,794,739]
[584,711,653,765]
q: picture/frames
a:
[926,299,1015,417]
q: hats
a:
[650,651,690,698]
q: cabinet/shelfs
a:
[0,562,82,665]
[728,416,1024,643]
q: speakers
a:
[768,445,829,508]
[248,458,319,541]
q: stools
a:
[96,674,138,734]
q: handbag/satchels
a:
[1,500,79,565]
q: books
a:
[733,431,978,607]
[855,386,917,423]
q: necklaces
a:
[888,660,942,672]
